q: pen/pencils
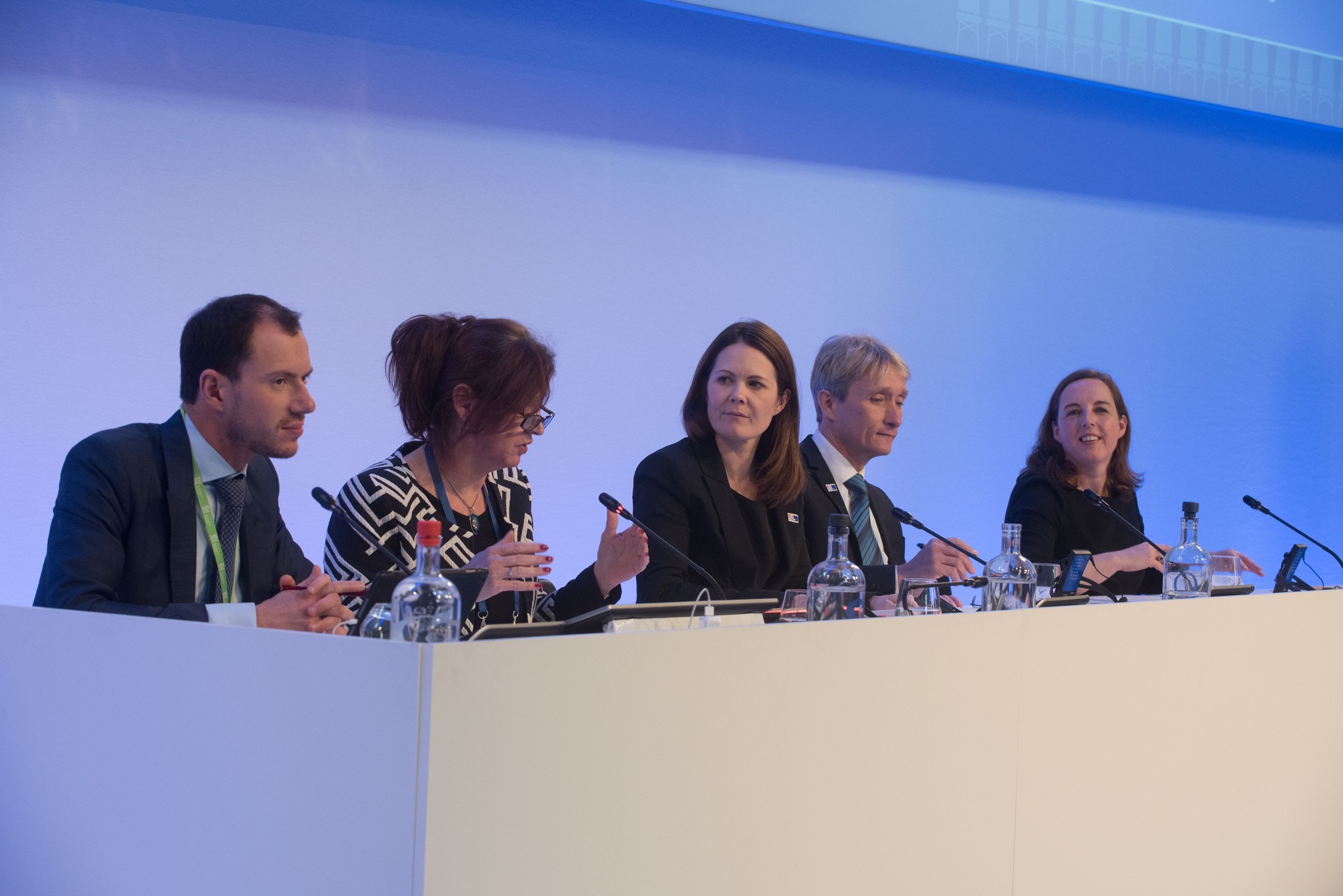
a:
[282,585,367,596]
[917,543,979,557]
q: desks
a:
[2,587,1343,896]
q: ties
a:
[843,473,884,566]
[210,477,247,603]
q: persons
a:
[30,294,366,636]
[633,321,919,624]
[798,335,979,608]
[1005,369,1264,597]
[322,313,651,640]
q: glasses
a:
[514,405,555,431]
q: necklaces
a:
[434,456,482,535]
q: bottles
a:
[389,521,461,642]
[1162,501,1213,600]
[806,514,865,621]
[981,523,1037,612]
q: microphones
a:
[1242,495,1343,569]
[598,492,728,600]
[902,576,988,612]
[1082,488,1192,592]
[892,507,987,566]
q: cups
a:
[779,589,807,622]
[1033,563,1061,605]
[1210,555,1243,587]
[894,578,941,616]
[359,602,391,639]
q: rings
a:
[635,550,641,559]
[1158,553,1164,562]
[509,566,513,578]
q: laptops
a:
[347,568,491,640]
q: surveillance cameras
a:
[312,488,411,576]
